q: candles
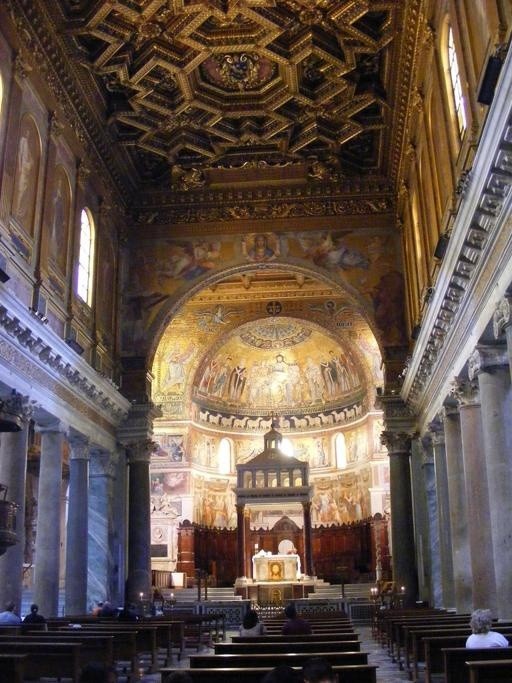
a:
[140,592,143,600]
[171,593,173,599]
[375,588,378,595]
[371,588,374,595]
[401,586,404,593]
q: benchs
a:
[1,610,228,682]
[161,610,380,682]
[373,609,511,683]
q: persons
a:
[79,663,119,683]
[23,604,46,624]
[281,606,313,635]
[238,610,266,637]
[270,563,283,580]
[264,666,304,683]
[301,656,339,683]
[91,601,114,617]
[0,600,20,624]
[163,672,192,682]
[465,608,509,649]
